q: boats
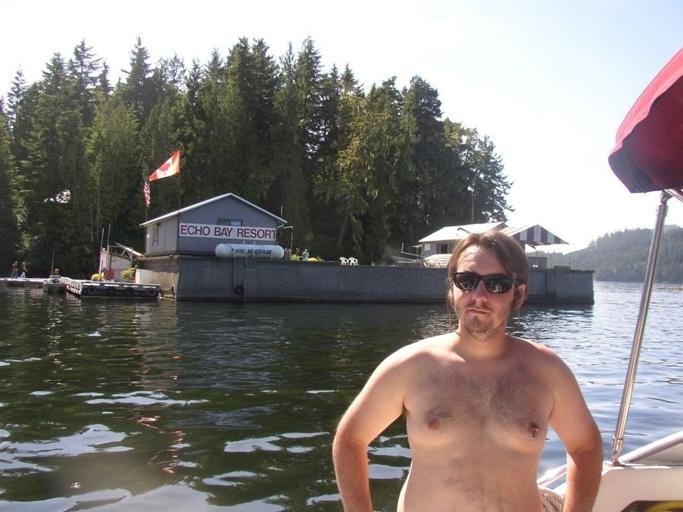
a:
[41,278,68,294]
[535,43,683,512]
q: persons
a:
[331,229,602,511]
[19,262,26,278]
[10,260,17,278]
[301,248,309,258]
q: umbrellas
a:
[607,43,683,196]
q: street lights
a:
[466,181,474,223]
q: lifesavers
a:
[235,285,244,295]
[87,286,155,297]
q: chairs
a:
[339,256,359,266]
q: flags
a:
[143,178,151,208]
[148,150,180,182]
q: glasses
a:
[453,271,522,294]
